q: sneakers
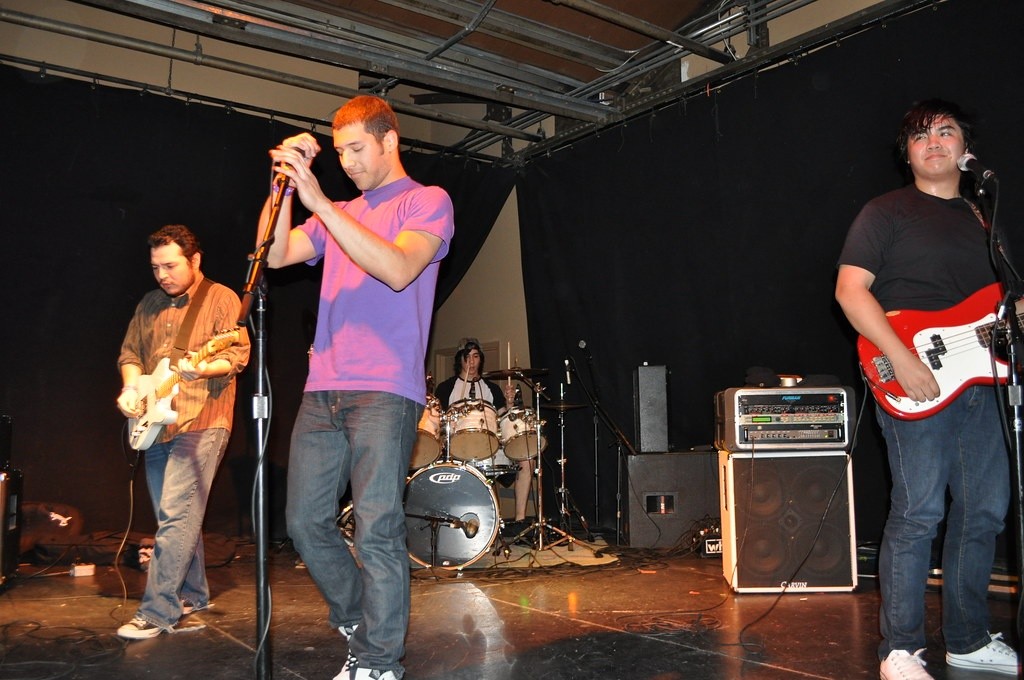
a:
[116,613,165,639]
[179,599,211,615]
[879,647,935,680]
[332,624,399,680]
[945,630,1018,676]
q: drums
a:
[444,397,501,462]
[465,444,518,476]
[500,405,549,461]
[410,393,446,469]
[400,460,501,570]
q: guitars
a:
[855,278,1024,420]
[128,324,243,451]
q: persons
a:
[436,337,537,521]
[255,94,455,680]
[114,222,253,639]
[833,90,1024,680]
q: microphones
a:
[957,154,1000,184]
[448,518,479,539]
[426,370,433,396]
[514,385,523,406]
[565,357,571,385]
[579,340,594,361]
[470,379,476,401]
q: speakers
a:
[633,366,668,452]
[0,469,23,580]
[630,452,722,548]
[719,451,857,592]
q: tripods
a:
[493,367,604,558]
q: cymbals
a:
[535,400,592,414]
[477,366,550,381]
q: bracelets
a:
[122,384,138,392]
[269,184,293,195]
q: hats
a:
[455,337,484,354]
